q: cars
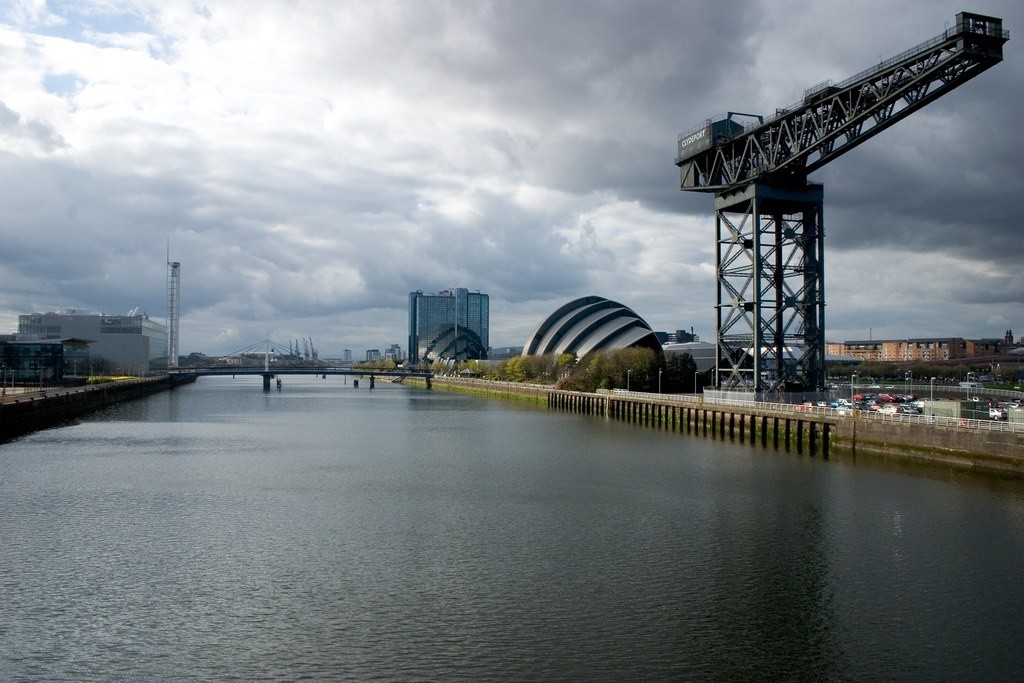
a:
[971,397,1024,420]
[803,394,923,414]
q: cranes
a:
[676,10,1009,392]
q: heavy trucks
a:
[926,400,990,425]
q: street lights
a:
[931,377,936,401]
[967,372,975,400]
[659,371,662,394]
[628,370,630,390]
[851,374,856,409]
[711,370,714,386]
[904,373,908,403]
[908,370,913,394]
[695,372,698,397]
